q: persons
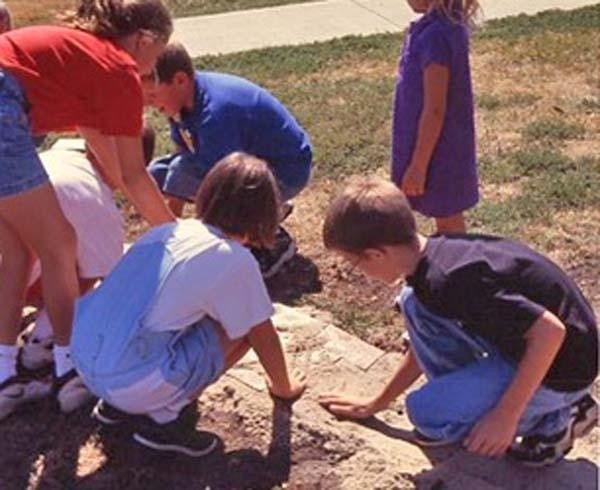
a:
[140,41,317,280]
[318,175,600,470]
[12,118,158,379]
[0,1,182,424]
[70,149,310,459]
[388,1,480,236]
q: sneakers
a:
[513,392,600,468]
[93,398,129,426]
[251,224,295,279]
[14,334,54,381]
[0,375,52,421]
[53,368,95,416]
[131,418,217,458]
